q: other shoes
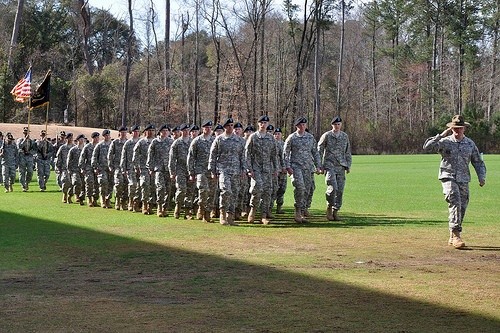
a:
[10,184,13,192]
[41,188,44,192]
[44,182,46,189]
[5,186,9,192]
[23,187,26,192]
[26,183,29,190]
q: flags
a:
[26,71,51,110]
[10,66,32,103]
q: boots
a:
[63,191,283,225]
[326,206,334,221]
[448,229,466,248]
[295,207,309,223]
[304,208,311,218]
[333,209,341,221]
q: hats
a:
[0,116,282,142]
[446,115,470,128]
[295,117,307,126]
[331,117,342,124]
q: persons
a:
[282,117,321,223]
[318,117,352,221]
[245,115,278,225]
[0,118,315,226]
[423,115,487,249]
